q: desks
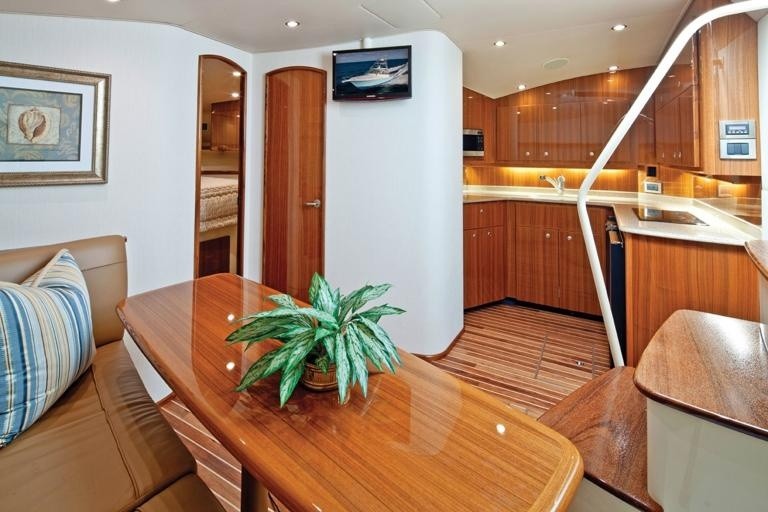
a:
[115,272,586,511]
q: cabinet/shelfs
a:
[464,201,608,319]
[495,102,582,167]
[653,0,761,176]
[582,98,631,168]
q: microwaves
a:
[463,128,484,156]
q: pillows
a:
[0,249,97,449]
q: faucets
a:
[539,174,566,195]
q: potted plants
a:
[225,269,408,411]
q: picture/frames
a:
[0,60,113,188]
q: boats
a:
[340,57,409,90]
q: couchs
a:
[0,232,228,512]
